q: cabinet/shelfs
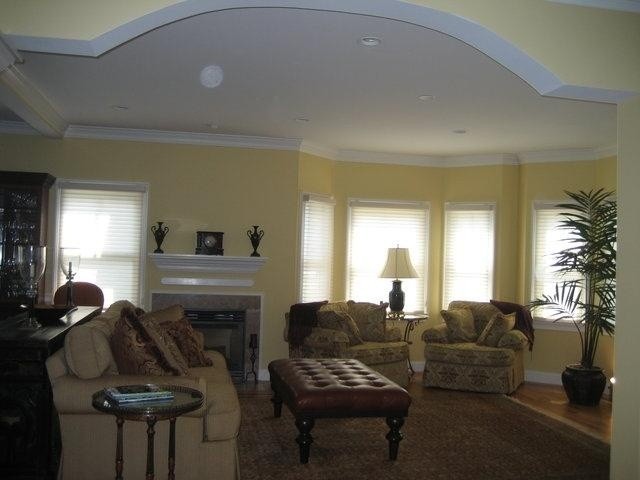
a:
[0,170,56,304]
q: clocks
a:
[195,230,224,256]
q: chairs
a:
[52,283,103,316]
[421,301,525,393]
[283,305,410,390]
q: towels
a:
[491,300,535,352]
[287,300,328,349]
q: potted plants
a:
[521,186,616,405]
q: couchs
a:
[45,300,240,480]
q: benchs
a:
[266,356,412,465]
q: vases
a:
[150,221,169,253]
[246,224,264,257]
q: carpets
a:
[235,378,610,480]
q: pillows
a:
[441,308,479,342]
[474,310,516,346]
[347,300,389,342]
[60,301,213,380]
[316,309,364,346]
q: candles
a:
[67,261,73,277]
[30,265,35,277]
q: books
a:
[107,382,172,396]
[105,393,172,404]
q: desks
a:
[91,384,206,480]
[383,313,429,375]
[0,303,106,480]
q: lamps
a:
[377,243,421,320]
